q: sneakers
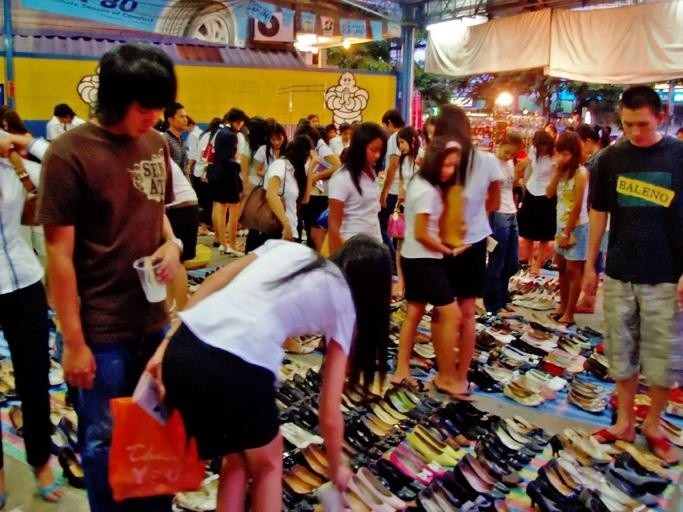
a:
[212,240,222,247]
[507,270,564,308]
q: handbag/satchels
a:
[11,149,41,226]
[108,390,206,501]
[386,201,407,239]
[487,178,503,214]
[237,162,286,233]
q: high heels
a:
[228,248,244,258]
[539,464,600,507]
[219,245,229,255]
[527,482,589,512]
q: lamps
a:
[490,81,534,119]
[423,13,495,45]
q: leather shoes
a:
[614,376,683,436]
[0,344,69,443]
[472,315,610,412]
[277,401,541,512]
[391,302,437,372]
[275,362,433,399]
[53,407,91,485]
[285,334,323,353]
[189,266,221,284]
[602,455,678,510]
[564,426,616,463]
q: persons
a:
[433,104,505,389]
[163,102,191,187]
[329,122,352,153]
[422,116,438,143]
[325,124,338,144]
[34,43,186,512]
[545,132,589,326]
[0,129,66,510]
[193,117,221,236]
[380,108,409,284]
[244,135,318,258]
[3,110,31,140]
[227,115,265,234]
[135,230,393,512]
[308,114,325,135]
[389,133,472,397]
[207,107,246,258]
[576,123,610,314]
[327,121,388,259]
[184,116,204,177]
[295,124,342,251]
[52,102,86,131]
[253,123,288,177]
[487,130,532,282]
[391,127,426,297]
[518,130,559,276]
[580,84,683,466]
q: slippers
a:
[437,385,477,401]
[648,435,680,468]
[589,427,635,445]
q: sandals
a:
[37,464,69,505]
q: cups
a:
[133,256,166,302]
